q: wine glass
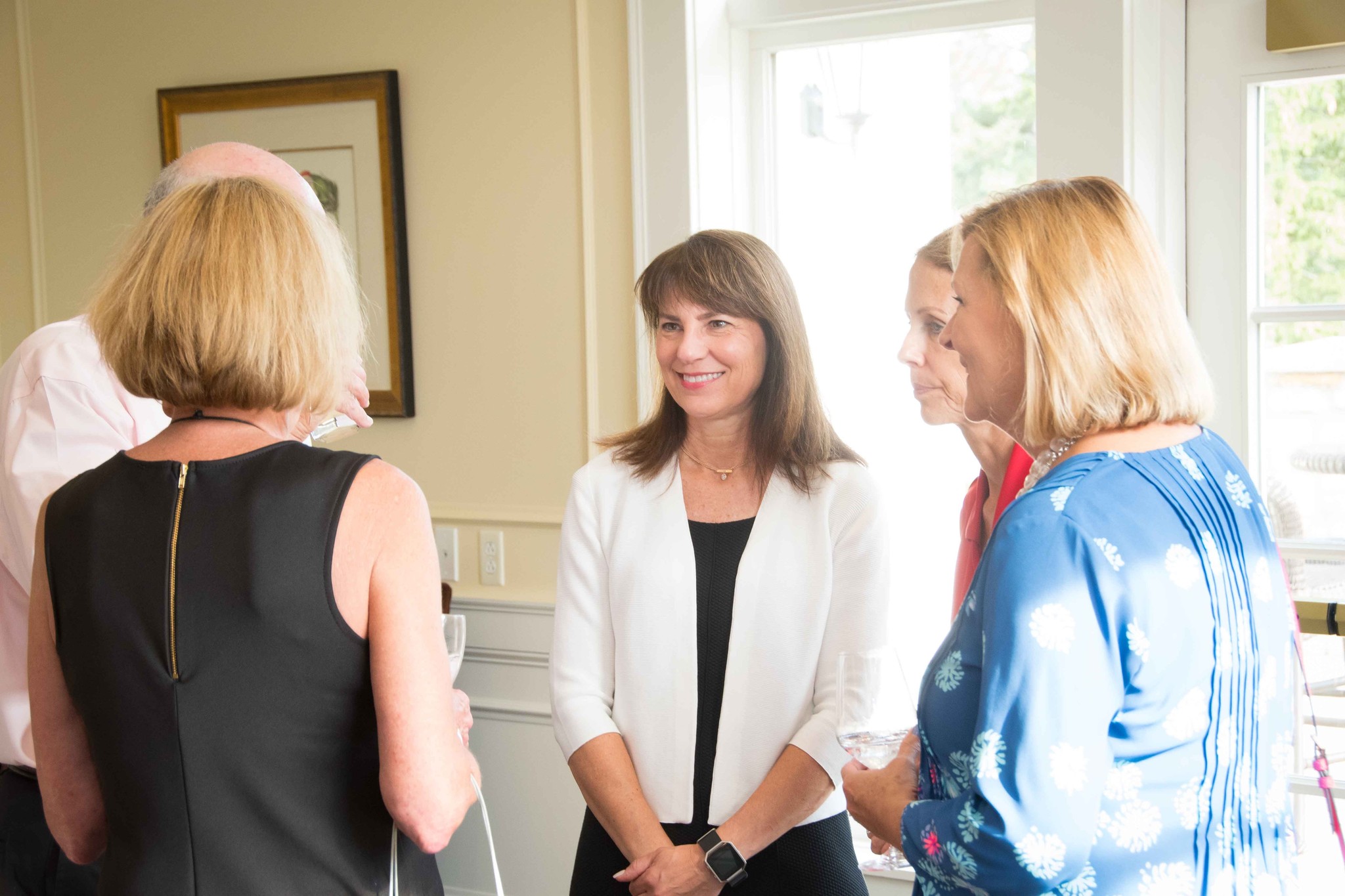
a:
[836,650,911,870]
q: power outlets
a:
[435,526,460,582]
[478,530,504,587]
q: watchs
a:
[697,828,749,888]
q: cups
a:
[443,615,465,679]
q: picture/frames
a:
[157,67,415,419]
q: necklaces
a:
[678,440,762,481]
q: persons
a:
[547,228,900,896]
[836,176,1304,896]
[0,140,487,896]
